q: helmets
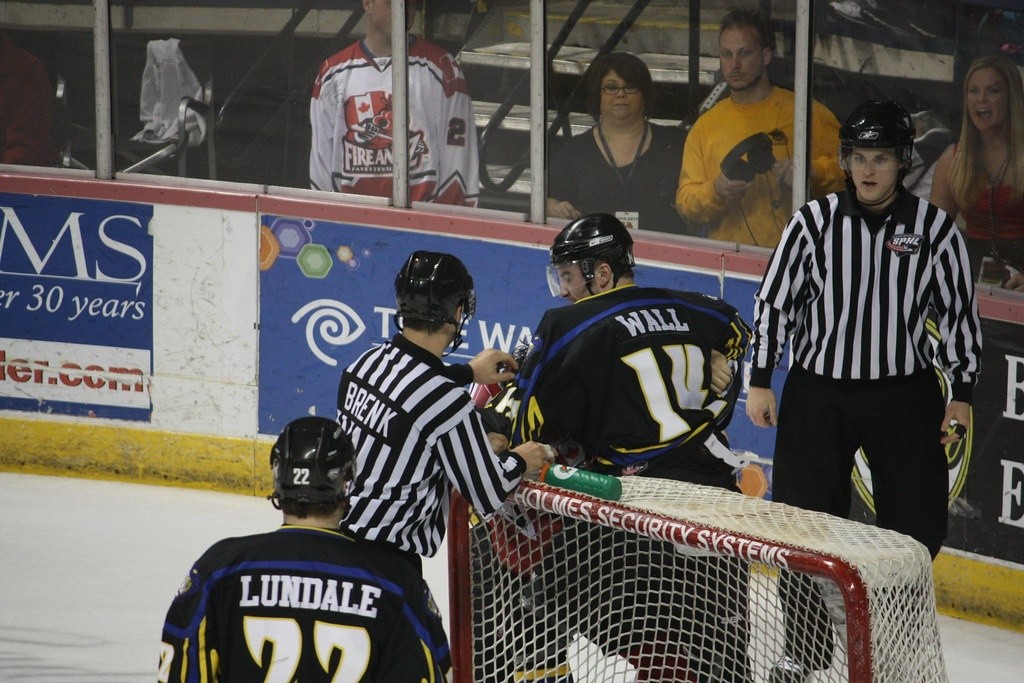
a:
[838,100,915,168]
[549,213,636,282]
[269,415,359,510]
[395,250,477,324]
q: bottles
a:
[540,464,622,501]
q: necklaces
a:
[980,156,1011,198]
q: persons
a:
[1,34,52,165]
[675,10,848,249]
[547,53,686,236]
[480,213,755,683]
[745,100,982,683]
[929,0,1024,292]
[309,0,480,208]
[334,251,558,579]
[157,416,451,683]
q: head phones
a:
[721,131,776,183]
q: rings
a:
[538,443,554,457]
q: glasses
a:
[601,85,640,95]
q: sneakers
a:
[769,653,815,683]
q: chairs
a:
[50,39,220,179]
